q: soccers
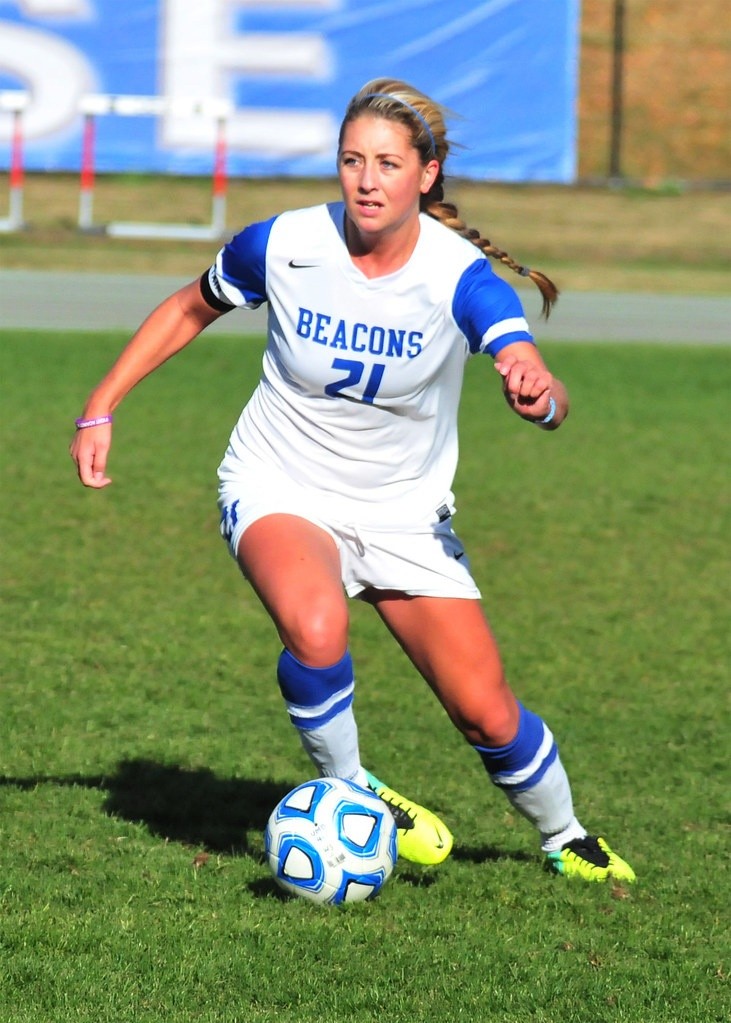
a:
[265,777,399,907]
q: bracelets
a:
[530,393,556,424]
[74,415,112,432]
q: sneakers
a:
[359,765,452,867]
[541,836,636,886]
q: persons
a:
[69,74,648,889]
